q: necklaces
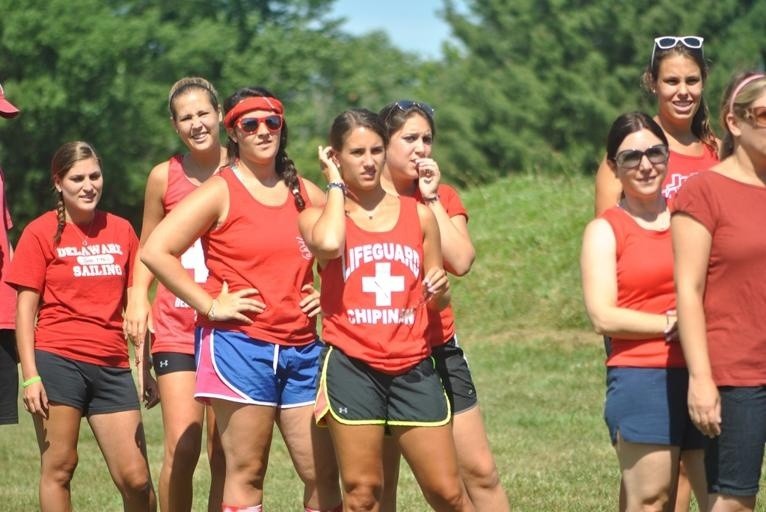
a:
[65,218,94,246]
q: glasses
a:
[735,107,766,127]
[382,101,436,122]
[649,36,705,74]
[608,143,670,170]
[232,113,284,134]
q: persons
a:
[595,36,724,512]
[123,78,227,512]
[3,143,161,512]
[0,82,20,425]
[581,111,710,512]
[378,102,512,511]
[670,71,766,512]
[140,86,343,512]
[298,109,477,512]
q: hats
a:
[0,86,20,118]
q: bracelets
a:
[22,376,41,388]
[423,194,440,205]
[327,182,345,192]
[135,358,153,370]
[209,300,217,321]
